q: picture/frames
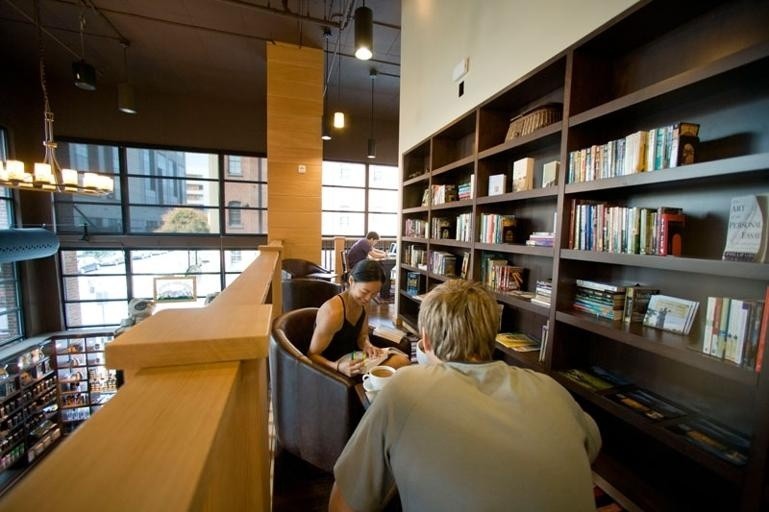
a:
[152,276,199,303]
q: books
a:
[373,324,407,344]
[390,243,397,253]
[569,122,701,184]
[568,199,684,256]
[339,345,410,375]
[403,158,560,363]
[722,193,769,264]
[571,277,769,374]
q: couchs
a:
[280,259,345,315]
[269,306,411,476]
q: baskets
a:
[505,108,554,142]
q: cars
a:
[76,249,169,273]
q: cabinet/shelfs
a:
[1,337,62,494]
[52,334,123,436]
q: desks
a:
[353,382,371,411]
[369,253,397,300]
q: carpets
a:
[372,292,395,305]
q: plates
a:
[363,379,373,394]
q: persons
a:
[367,324,412,359]
[307,262,415,385]
[335,359,342,373]
[326,279,604,512]
[347,231,388,267]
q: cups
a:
[361,366,395,390]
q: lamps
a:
[73,14,99,93]
[353,1,374,63]
[117,47,140,116]
[367,69,377,159]
[334,33,347,133]
[0,54,115,197]
[321,28,333,141]
